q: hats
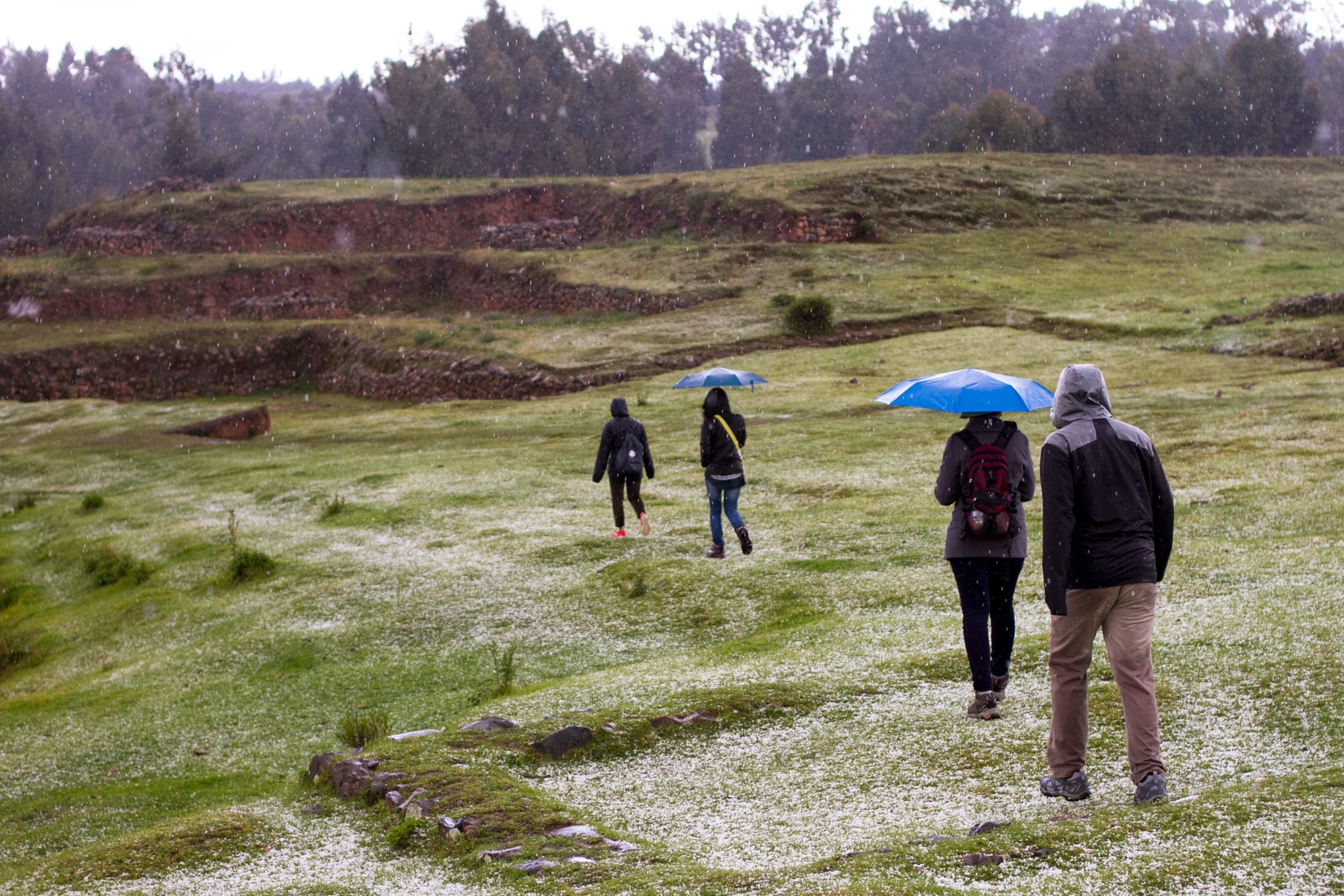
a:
[960,412,992,418]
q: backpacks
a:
[955,419,1021,542]
[611,418,643,475]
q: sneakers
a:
[988,672,1009,700]
[735,525,753,555]
[1133,771,1167,805]
[706,545,724,558]
[611,530,625,538]
[966,691,1002,718]
[1040,769,1092,801]
[640,513,652,536]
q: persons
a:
[1037,364,1176,807]
[934,411,1036,719]
[592,397,655,539]
[700,387,753,558]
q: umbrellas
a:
[670,365,770,392]
[872,367,1056,411]
